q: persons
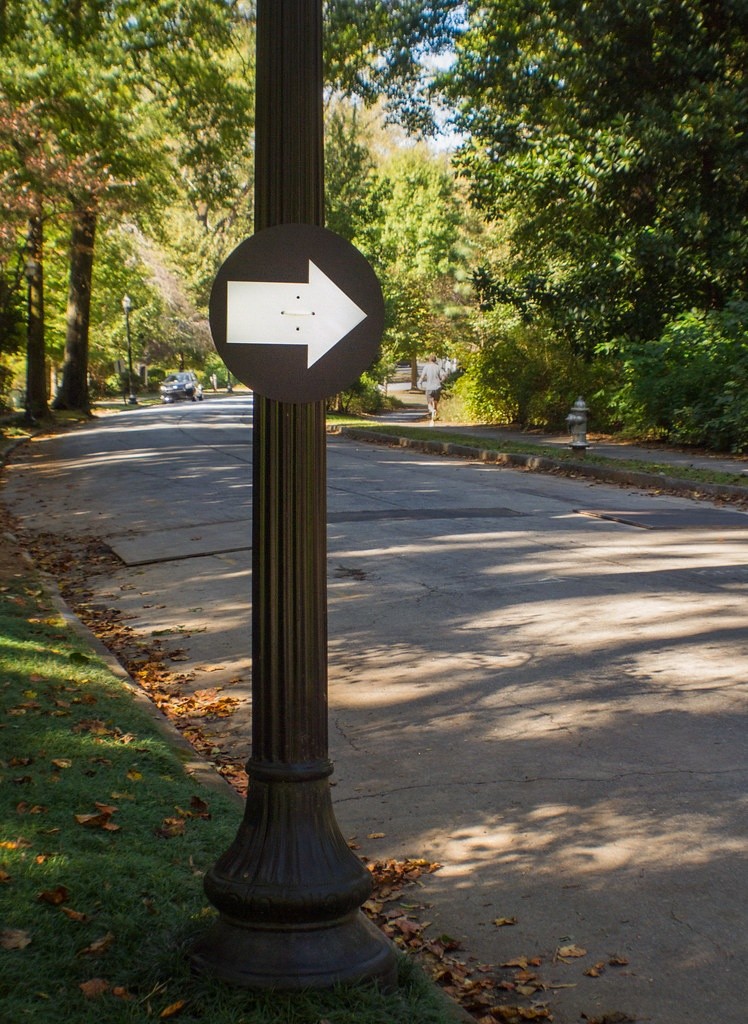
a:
[418,355,446,424]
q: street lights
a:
[121,292,138,405]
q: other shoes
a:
[433,410,438,421]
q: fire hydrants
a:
[565,399,592,457]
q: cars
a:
[160,372,203,404]
